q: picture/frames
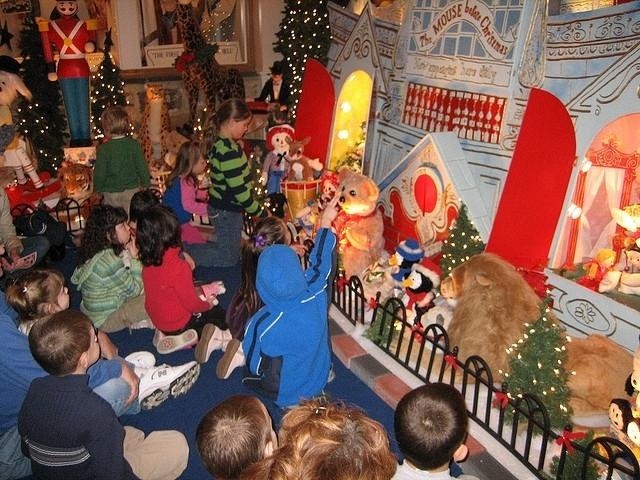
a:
[90,0,257,86]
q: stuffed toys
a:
[437,243,639,462]
[137,82,172,165]
[173,2,244,142]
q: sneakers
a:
[152,327,165,349]
[138,361,200,411]
[125,351,156,369]
[38,243,66,264]
[157,329,198,354]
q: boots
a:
[194,324,232,364]
[216,339,246,379]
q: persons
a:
[1,99,483,480]
[36,0,101,147]
[245,58,290,131]
